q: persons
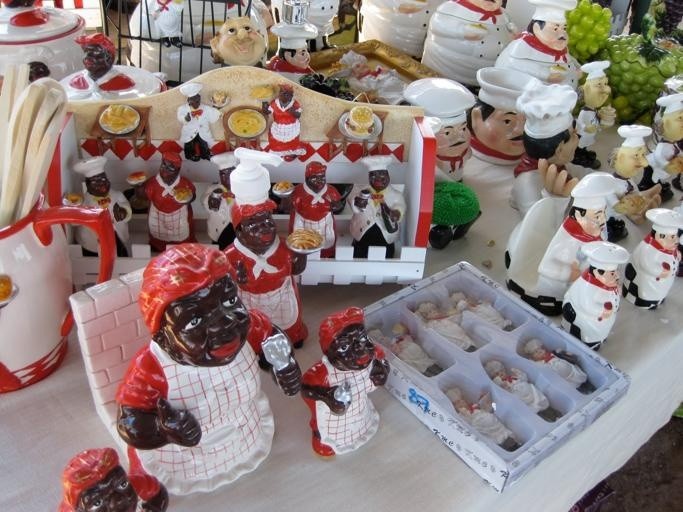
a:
[561,239,630,352]
[340,51,409,106]
[444,386,518,451]
[358,0,442,59]
[368,326,442,378]
[134,151,196,250]
[301,306,390,456]
[641,91,683,196]
[262,83,304,153]
[209,16,268,68]
[604,124,652,239]
[494,0,581,91]
[116,242,303,496]
[523,338,596,396]
[485,359,563,422]
[574,60,611,164]
[621,207,681,311]
[58,446,170,512]
[403,78,476,183]
[224,200,310,368]
[288,160,346,256]
[349,155,407,257]
[267,20,315,79]
[450,292,514,334]
[270,0,339,51]
[470,68,542,164]
[149,0,184,47]
[69,156,133,258]
[76,32,115,73]
[417,302,477,354]
[127,0,268,88]
[509,84,578,217]
[536,172,620,298]
[203,151,239,247]
[422,0,521,92]
[175,81,221,161]
